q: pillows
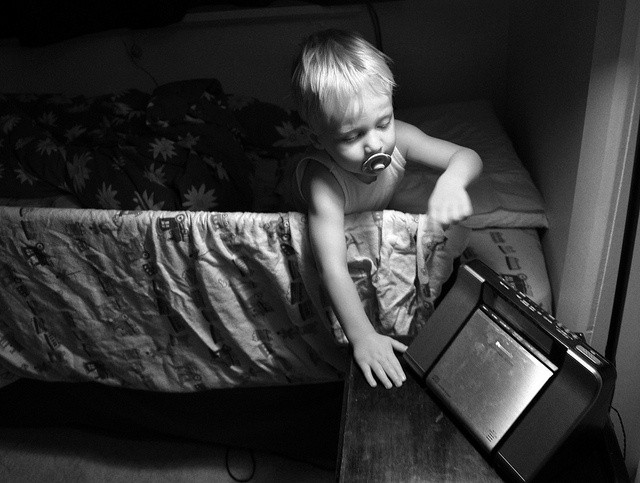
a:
[395,104,551,230]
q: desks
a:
[336,335,634,482]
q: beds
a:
[1,78,554,396]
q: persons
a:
[296,34,485,389]
